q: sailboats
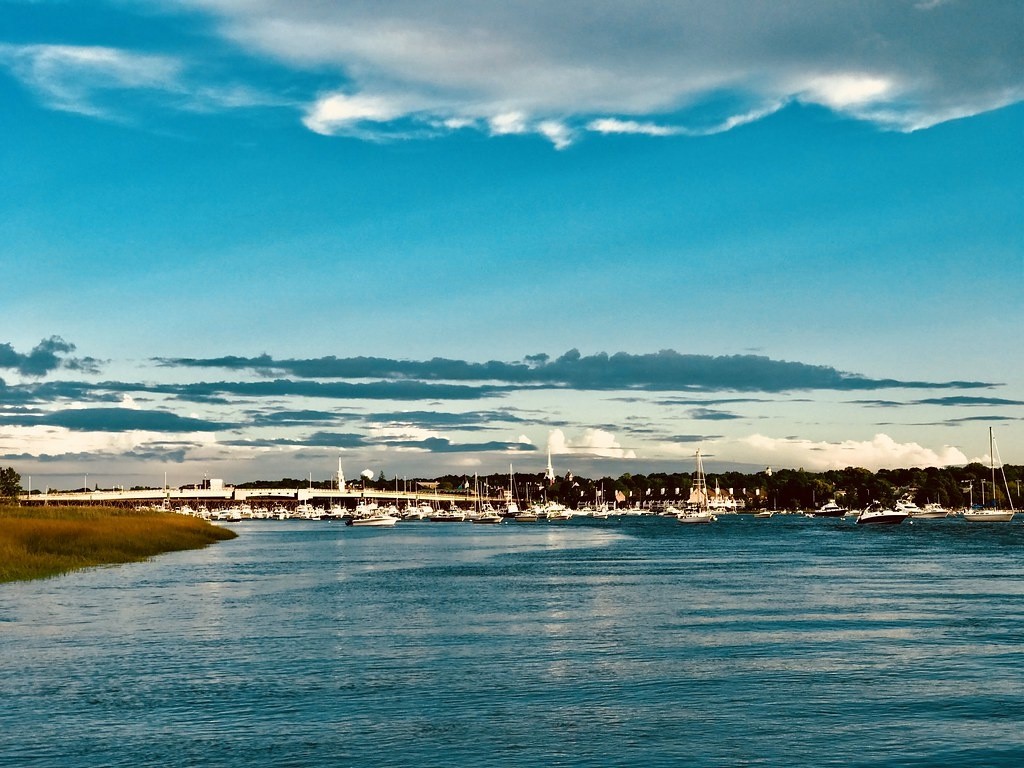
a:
[963,427,1015,522]
[677,447,718,523]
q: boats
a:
[754,511,774,518]
[663,512,678,517]
[131,463,657,521]
[814,499,848,517]
[429,498,467,521]
[907,502,948,518]
[345,508,401,527]
[514,509,538,522]
[854,499,909,524]
[473,503,504,523]
